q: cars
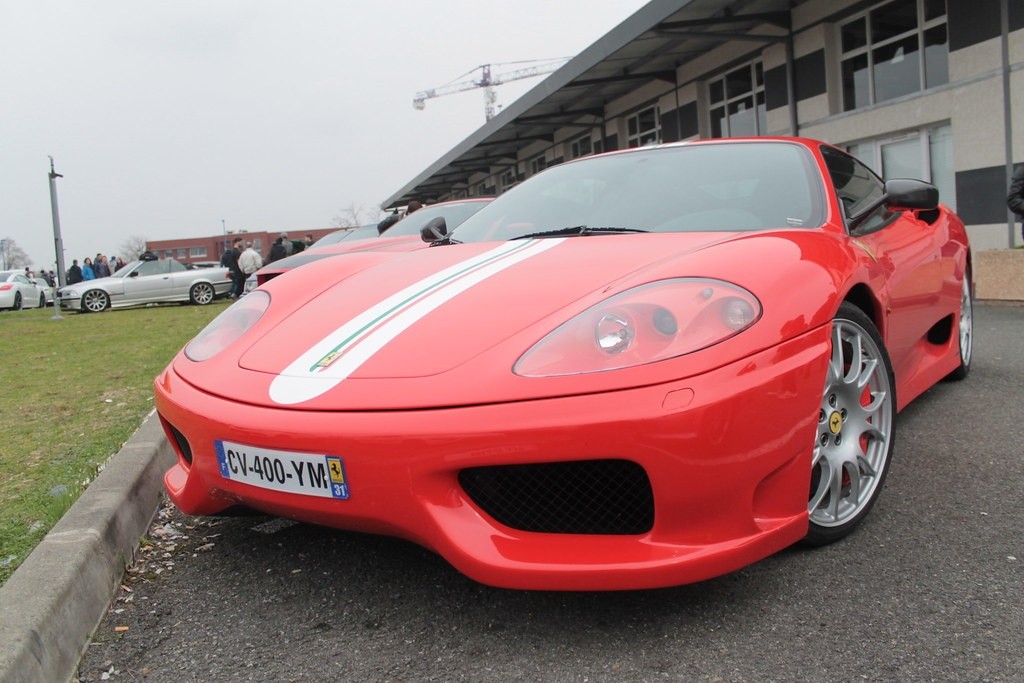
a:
[182,261,224,272]
[55,255,234,313]
[32,277,55,306]
[0,270,49,311]
[148,137,975,592]
[308,224,381,250]
[239,196,497,300]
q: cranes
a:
[411,56,574,122]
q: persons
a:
[377,201,423,234]
[66,253,127,283]
[221,233,314,300]
[49,271,56,287]
[25,267,35,278]
[40,270,51,286]
[139,245,159,260]
